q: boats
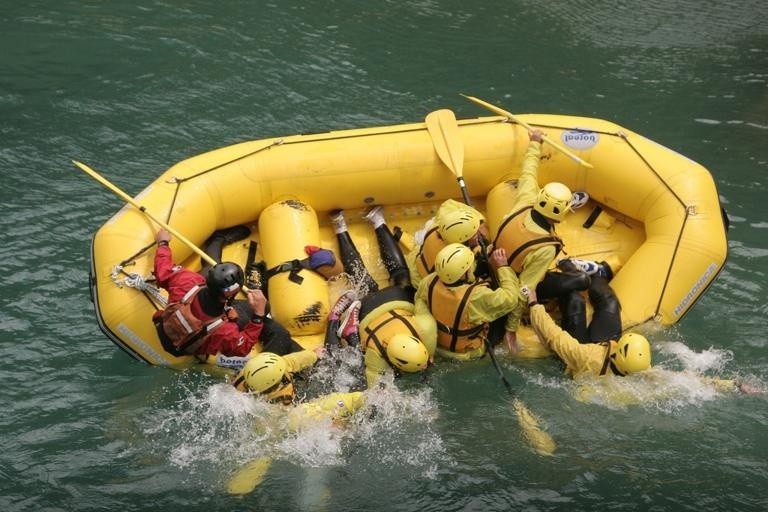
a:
[87,111,731,378]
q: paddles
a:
[73,158,251,297]
[425,108,499,289]
[464,92,593,168]
[484,336,556,455]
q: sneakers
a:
[363,204,383,220]
[572,191,589,209]
[326,291,363,339]
[557,257,597,277]
[329,210,344,222]
[206,226,251,245]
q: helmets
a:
[207,262,244,298]
[534,183,572,222]
[616,332,650,375]
[435,210,481,284]
[387,335,428,373]
[244,352,287,392]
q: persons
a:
[527,260,763,397]
[418,244,520,360]
[329,204,437,392]
[299,290,368,396]
[230,348,387,432]
[153,224,267,356]
[493,129,601,355]
[407,197,491,290]
